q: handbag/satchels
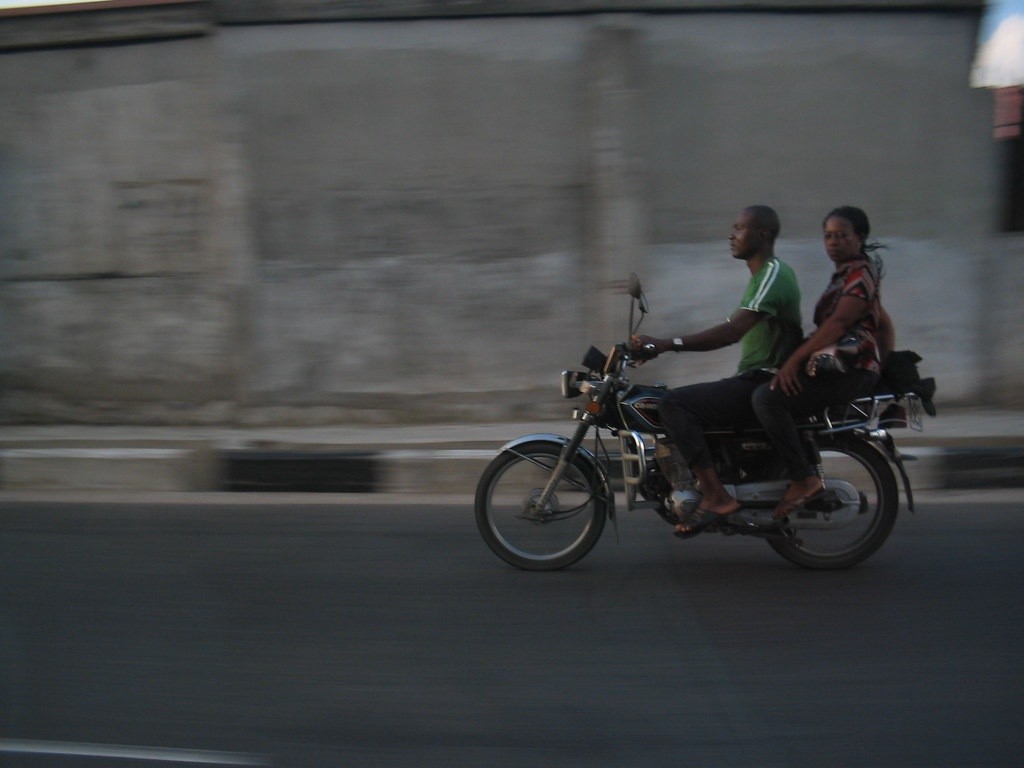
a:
[805,343,858,378]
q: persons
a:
[752,208,895,509]
[633,205,805,538]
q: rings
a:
[636,338,640,342]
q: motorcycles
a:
[473,269,937,573]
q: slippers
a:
[772,489,827,519]
[673,499,740,538]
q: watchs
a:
[672,336,683,352]
